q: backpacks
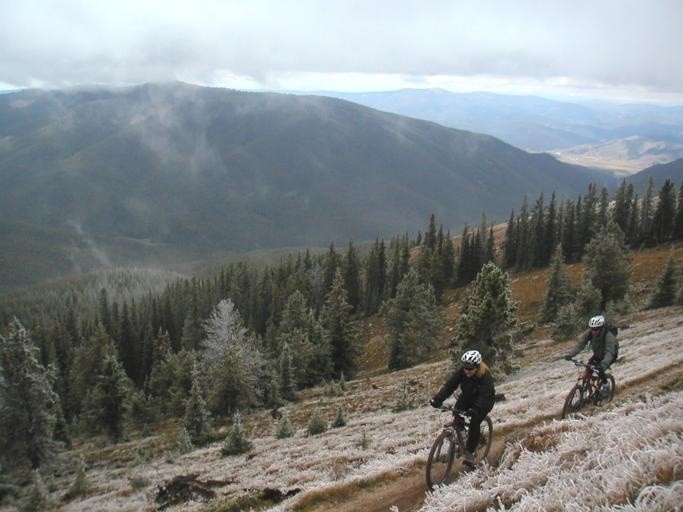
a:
[589,323,619,358]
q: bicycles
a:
[426,400,493,491]
[561,359,615,419]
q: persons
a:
[563,315,619,399]
[428,349,496,467]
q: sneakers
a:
[602,382,609,398]
[462,455,474,465]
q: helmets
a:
[588,315,605,329]
[460,350,482,365]
